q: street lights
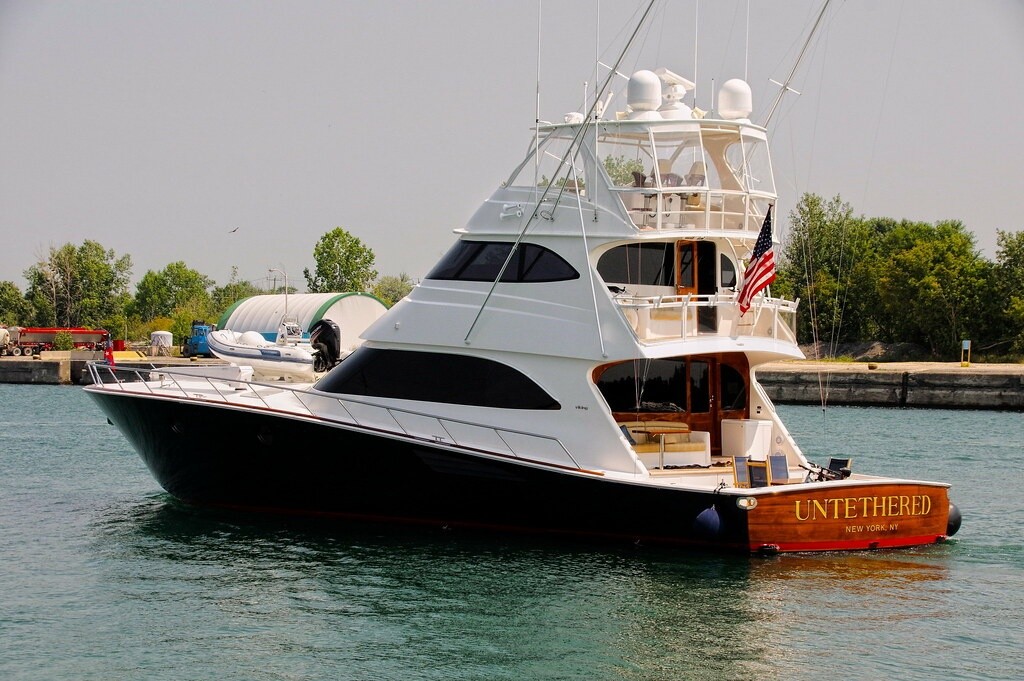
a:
[267,268,288,317]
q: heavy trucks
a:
[0,326,109,357]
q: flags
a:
[104,348,117,373]
[737,208,777,318]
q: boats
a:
[86,2,962,562]
[206,323,315,374]
